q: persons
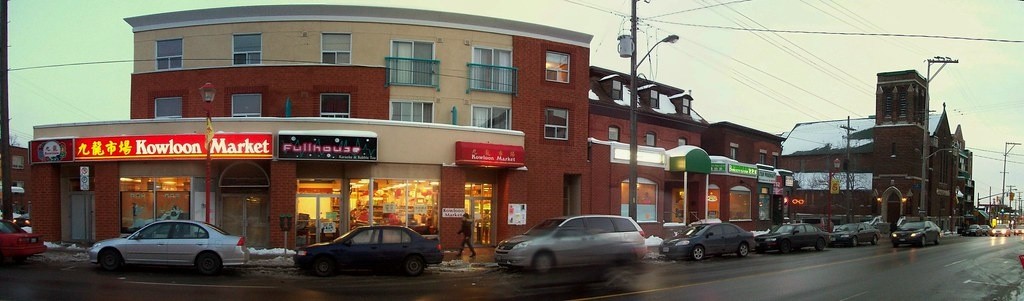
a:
[457,213,476,257]
[476,210,490,245]
[890,220,897,237]
[382,213,402,226]
[350,208,358,220]
[831,222,834,231]
[359,209,369,222]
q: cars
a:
[89,220,250,276]
[659,223,754,261]
[891,221,941,247]
[0,219,48,264]
[992,224,1011,236]
[980,225,991,236]
[755,223,830,254]
[1014,225,1024,235]
[293,225,442,277]
[962,224,982,236]
[830,223,879,247]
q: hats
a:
[463,213,470,219]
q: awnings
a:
[980,211,989,219]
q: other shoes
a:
[469,253,476,257]
[456,254,461,257]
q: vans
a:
[494,214,647,276]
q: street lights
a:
[919,148,957,222]
[828,158,840,233]
[199,83,219,223]
[628,34,679,224]
